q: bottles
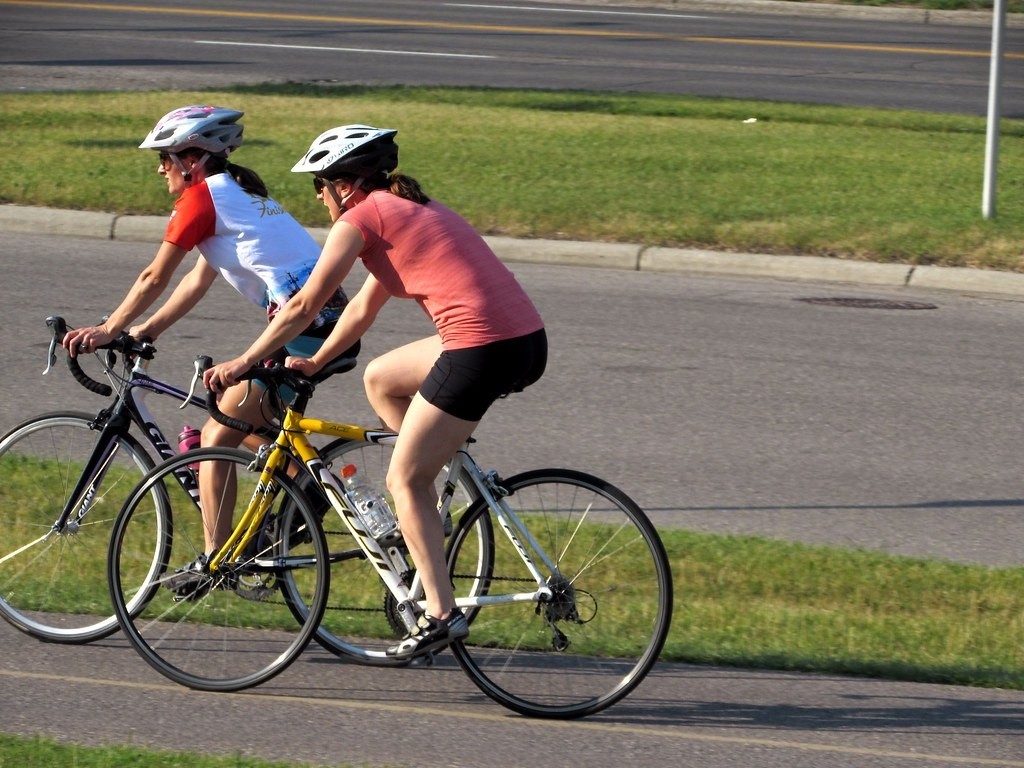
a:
[341,464,397,538]
[177,425,201,487]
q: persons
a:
[63,107,361,595]
[202,124,550,667]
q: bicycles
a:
[1,315,497,668]
[105,351,676,719]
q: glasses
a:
[314,178,342,194]
[159,154,186,169]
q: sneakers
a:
[395,606,470,658]
[377,508,452,547]
[159,550,238,595]
[287,471,345,542]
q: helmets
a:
[138,105,244,158]
[290,124,398,178]
